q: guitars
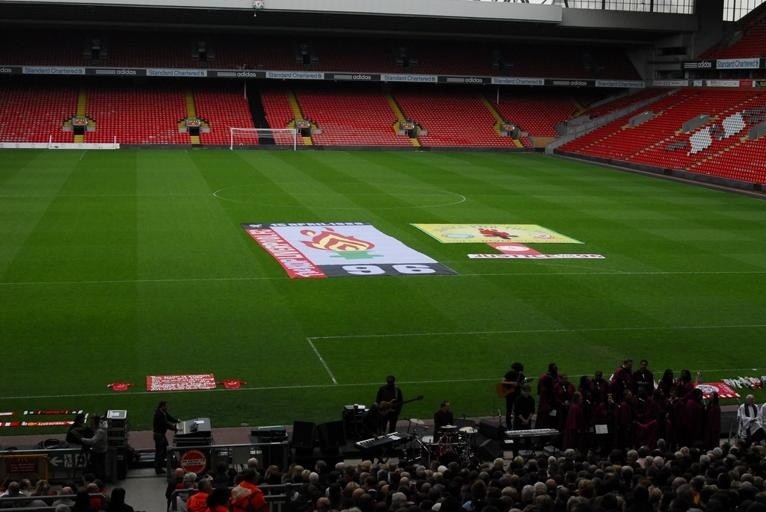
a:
[379,395,424,414]
[497,378,533,399]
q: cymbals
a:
[461,426,478,433]
[441,424,456,428]
[410,418,424,425]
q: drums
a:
[449,441,469,459]
[431,443,447,457]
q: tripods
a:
[71,444,95,481]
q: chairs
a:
[706,6,766,63]
[641,85,766,187]
[577,152,641,167]
[0,24,641,152]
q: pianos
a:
[355,431,407,449]
[505,429,560,438]
